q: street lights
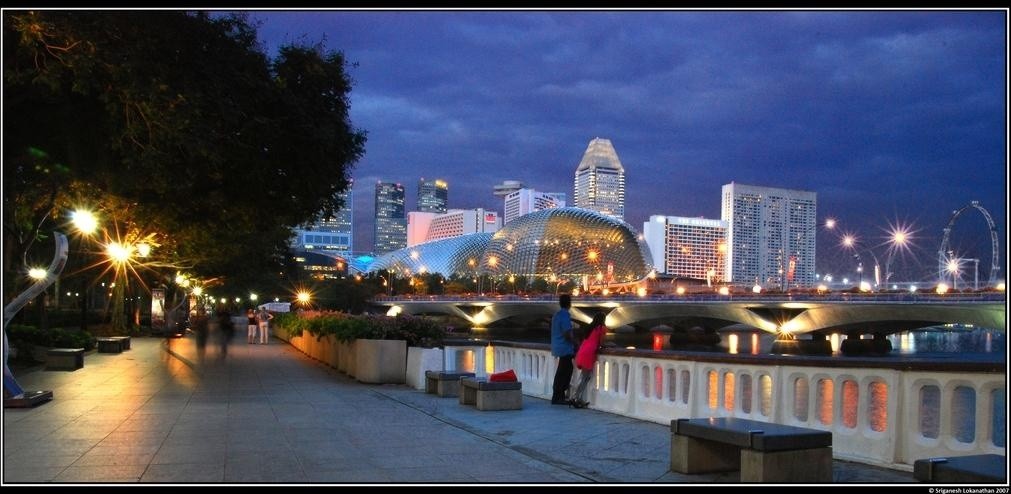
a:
[583,246,601,292]
[390,250,420,294]
[795,214,840,285]
[483,253,515,295]
[884,215,924,292]
[57,197,107,331]
[838,228,882,290]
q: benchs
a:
[915,454,1004,483]
[425,370,522,411]
[670,417,833,482]
[46,348,85,371]
[98,337,130,353]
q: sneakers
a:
[552,397,590,408]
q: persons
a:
[549,292,582,405]
[568,311,618,409]
[153,299,275,366]
